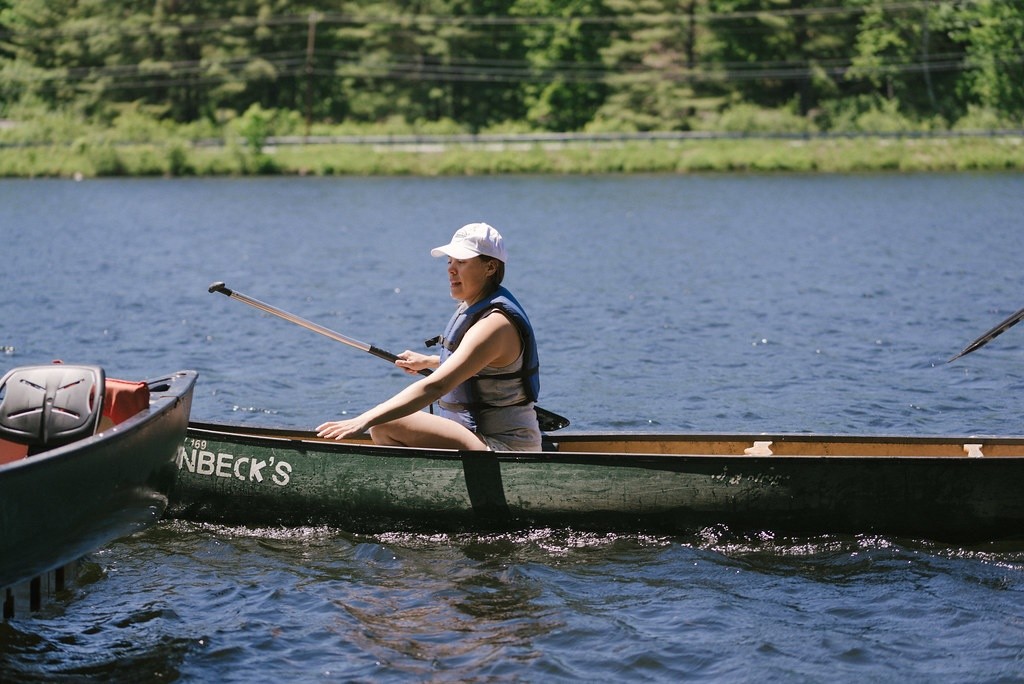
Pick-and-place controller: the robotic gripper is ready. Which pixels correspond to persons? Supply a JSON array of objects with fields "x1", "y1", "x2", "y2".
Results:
[{"x1": 315, "y1": 223, "x2": 544, "y2": 451}]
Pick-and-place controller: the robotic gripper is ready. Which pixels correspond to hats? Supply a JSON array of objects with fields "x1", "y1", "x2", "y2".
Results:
[{"x1": 430, "y1": 221, "x2": 508, "y2": 265}]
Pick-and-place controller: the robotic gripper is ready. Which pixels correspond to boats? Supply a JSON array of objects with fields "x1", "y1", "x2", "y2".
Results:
[
  {"x1": 170, "y1": 423, "x2": 1023, "y2": 555},
  {"x1": 0, "y1": 369, "x2": 199, "y2": 596}
]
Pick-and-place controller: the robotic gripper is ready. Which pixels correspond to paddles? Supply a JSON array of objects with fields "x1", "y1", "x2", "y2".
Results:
[
  {"x1": 208, "y1": 282, "x2": 571, "y2": 432},
  {"x1": 947, "y1": 308, "x2": 1024, "y2": 364}
]
